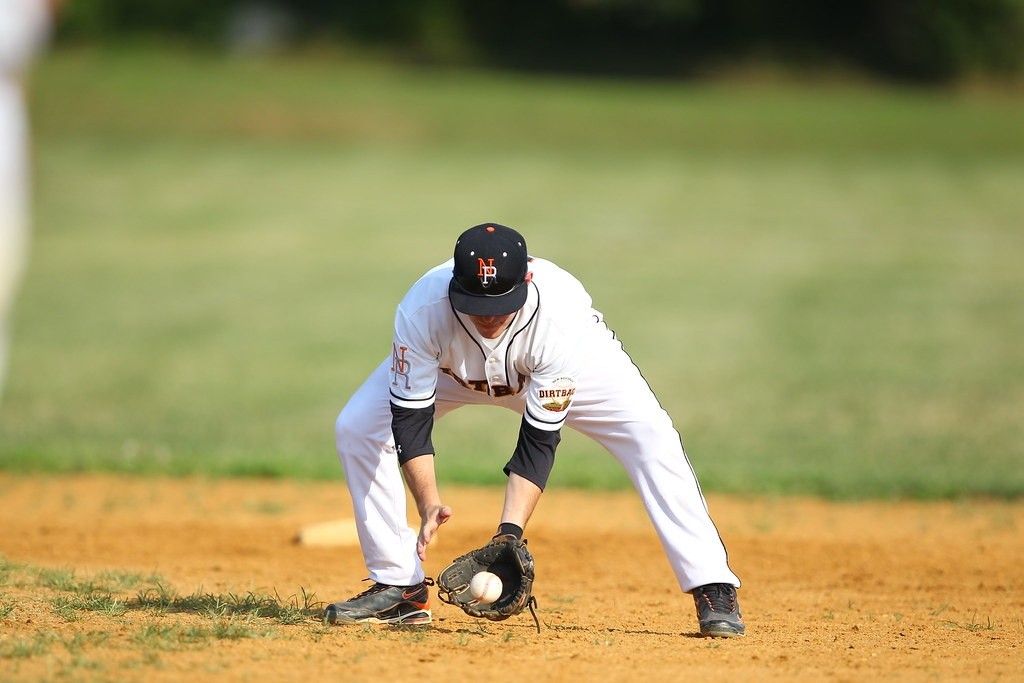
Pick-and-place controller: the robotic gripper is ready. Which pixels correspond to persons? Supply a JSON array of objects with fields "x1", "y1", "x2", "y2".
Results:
[{"x1": 325, "y1": 223, "x2": 746, "y2": 638}]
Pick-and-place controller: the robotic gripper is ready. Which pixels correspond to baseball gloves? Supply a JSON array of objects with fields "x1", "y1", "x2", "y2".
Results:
[{"x1": 436, "y1": 533, "x2": 541, "y2": 633}]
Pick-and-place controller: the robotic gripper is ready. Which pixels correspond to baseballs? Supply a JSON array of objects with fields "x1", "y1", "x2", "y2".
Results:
[{"x1": 470, "y1": 571, "x2": 504, "y2": 604}]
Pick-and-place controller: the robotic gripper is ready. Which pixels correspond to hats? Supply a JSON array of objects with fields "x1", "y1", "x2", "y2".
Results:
[{"x1": 450, "y1": 221, "x2": 528, "y2": 316}]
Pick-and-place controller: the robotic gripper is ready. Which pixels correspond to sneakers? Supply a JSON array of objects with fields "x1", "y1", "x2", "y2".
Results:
[
  {"x1": 323, "y1": 580, "x2": 431, "y2": 627},
  {"x1": 692, "y1": 583, "x2": 745, "y2": 637}
]
